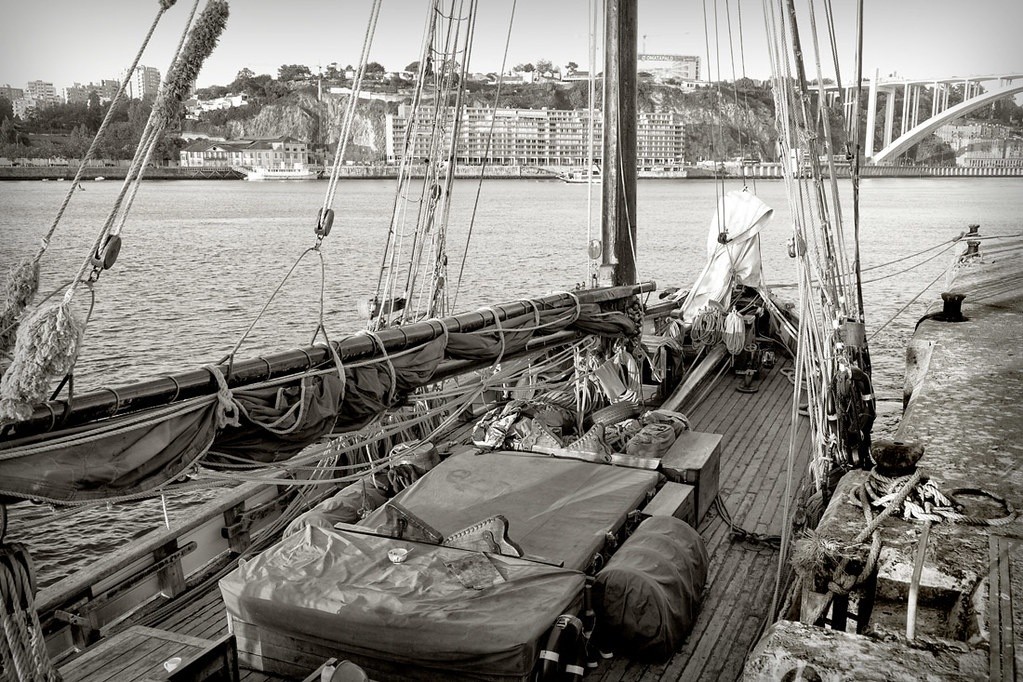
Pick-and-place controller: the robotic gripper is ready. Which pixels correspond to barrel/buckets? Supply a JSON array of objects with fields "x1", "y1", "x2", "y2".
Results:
[{"x1": 390, "y1": 440, "x2": 442, "y2": 477}]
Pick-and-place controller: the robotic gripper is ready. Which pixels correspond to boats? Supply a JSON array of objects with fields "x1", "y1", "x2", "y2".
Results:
[
  {"x1": 242, "y1": 165, "x2": 323, "y2": 182},
  {"x1": 555, "y1": 161, "x2": 602, "y2": 184}
]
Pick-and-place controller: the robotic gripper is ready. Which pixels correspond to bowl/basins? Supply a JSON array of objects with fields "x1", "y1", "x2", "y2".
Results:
[
  {"x1": 387, "y1": 548, "x2": 408, "y2": 562},
  {"x1": 329, "y1": 660, "x2": 369, "y2": 682}
]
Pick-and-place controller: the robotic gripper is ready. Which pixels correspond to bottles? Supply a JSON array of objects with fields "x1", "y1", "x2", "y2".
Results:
[{"x1": 321, "y1": 661, "x2": 336, "y2": 682}]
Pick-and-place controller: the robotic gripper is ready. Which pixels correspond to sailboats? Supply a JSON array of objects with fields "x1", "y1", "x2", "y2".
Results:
[{"x1": 0, "y1": 0, "x2": 920, "y2": 681}]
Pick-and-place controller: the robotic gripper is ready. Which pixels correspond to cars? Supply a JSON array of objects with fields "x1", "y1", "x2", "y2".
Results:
[{"x1": 48, "y1": 158, "x2": 69, "y2": 167}]
